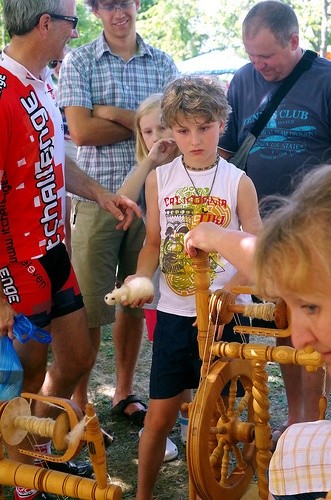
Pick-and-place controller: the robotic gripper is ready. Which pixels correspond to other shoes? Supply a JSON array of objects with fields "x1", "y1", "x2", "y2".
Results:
[
  {"x1": 179, "y1": 410, "x2": 189, "y2": 446},
  {"x1": 270, "y1": 424, "x2": 287, "y2": 452},
  {"x1": 138, "y1": 427, "x2": 178, "y2": 461},
  {"x1": 41, "y1": 459, "x2": 93, "y2": 479}
]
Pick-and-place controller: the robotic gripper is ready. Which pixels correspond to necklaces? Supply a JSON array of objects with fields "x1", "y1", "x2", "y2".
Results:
[{"x1": 181, "y1": 153, "x2": 220, "y2": 213}]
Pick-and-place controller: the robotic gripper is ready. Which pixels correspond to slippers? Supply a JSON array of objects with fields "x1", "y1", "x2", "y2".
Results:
[{"x1": 111, "y1": 394, "x2": 148, "y2": 427}]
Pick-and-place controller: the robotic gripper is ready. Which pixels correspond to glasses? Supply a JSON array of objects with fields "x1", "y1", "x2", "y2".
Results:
[
  {"x1": 97, "y1": 0, "x2": 135, "y2": 12},
  {"x1": 35, "y1": 12, "x2": 78, "y2": 29},
  {"x1": 50, "y1": 60, "x2": 62, "y2": 68}
]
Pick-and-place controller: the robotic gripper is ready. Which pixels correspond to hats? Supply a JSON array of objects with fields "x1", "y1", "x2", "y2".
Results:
[{"x1": 269, "y1": 420, "x2": 331, "y2": 496}]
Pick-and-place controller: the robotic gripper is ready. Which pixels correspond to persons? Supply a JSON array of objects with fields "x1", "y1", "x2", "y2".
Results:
[
  {"x1": 119, "y1": 77, "x2": 264, "y2": 500},
  {"x1": 184, "y1": 164, "x2": 331, "y2": 374},
  {"x1": 54, "y1": 0, "x2": 181, "y2": 452},
  {"x1": 114, "y1": 93, "x2": 194, "y2": 461},
  {"x1": 218, "y1": 0, "x2": 331, "y2": 453},
  {"x1": 0, "y1": 0, "x2": 141, "y2": 500},
  {"x1": 49, "y1": 46, "x2": 78, "y2": 260}
]
